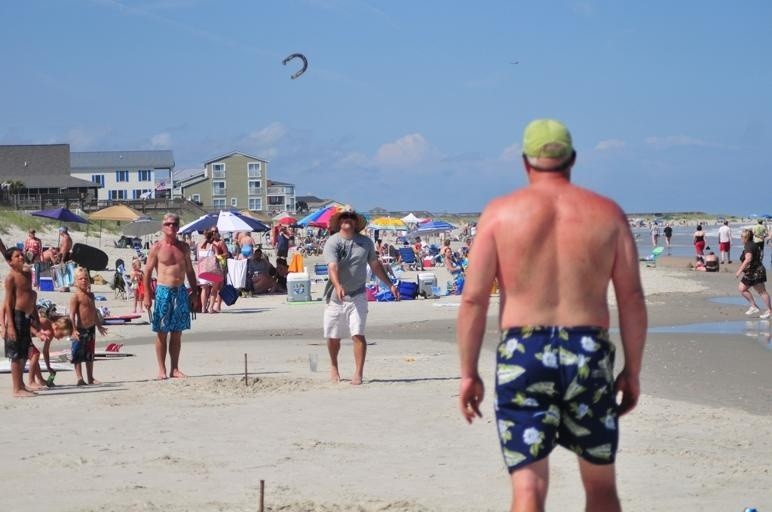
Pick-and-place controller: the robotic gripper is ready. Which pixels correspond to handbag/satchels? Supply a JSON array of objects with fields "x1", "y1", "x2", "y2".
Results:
[
  {"x1": 219, "y1": 284, "x2": 240, "y2": 305},
  {"x1": 197, "y1": 254, "x2": 224, "y2": 282},
  {"x1": 24, "y1": 251, "x2": 34, "y2": 263}
]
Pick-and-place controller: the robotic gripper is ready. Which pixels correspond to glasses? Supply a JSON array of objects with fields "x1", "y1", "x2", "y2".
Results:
[
  {"x1": 212, "y1": 230, "x2": 218, "y2": 233},
  {"x1": 163, "y1": 222, "x2": 180, "y2": 226},
  {"x1": 341, "y1": 212, "x2": 356, "y2": 220}
]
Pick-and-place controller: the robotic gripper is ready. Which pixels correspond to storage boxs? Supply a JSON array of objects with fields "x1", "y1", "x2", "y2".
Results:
[
  {"x1": 362, "y1": 272, "x2": 439, "y2": 303},
  {"x1": 38, "y1": 276, "x2": 55, "y2": 292},
  {"x1": 287, "y1": 271, "x2": 311, "y2": 303}
]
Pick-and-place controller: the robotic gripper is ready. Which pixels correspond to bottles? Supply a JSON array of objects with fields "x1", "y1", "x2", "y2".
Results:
[{"x1": 46, "y1": 372, "x2": 56, "y2": 386}]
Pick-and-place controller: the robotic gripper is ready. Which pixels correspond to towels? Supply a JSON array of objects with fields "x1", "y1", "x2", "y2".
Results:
[{"x1": 227, "y1": 259, "x2": 248, "y2": 291}]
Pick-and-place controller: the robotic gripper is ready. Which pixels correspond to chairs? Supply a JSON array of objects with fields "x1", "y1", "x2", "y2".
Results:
[
  {"x1": 112, "y1": 259, "x2": 213, "y2": 320},
  {"x1": 378, "y1": 240, "x2": 444, "y2": 269},
  {"x1": 225, "y1": 257, "x2": 253, "y2": 298}
]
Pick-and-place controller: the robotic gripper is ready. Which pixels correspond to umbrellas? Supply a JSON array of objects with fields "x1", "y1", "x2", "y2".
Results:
[
  {"x1": 237, "y1": 202, "x2": 368, "y2": 240},
  {"x1": 172, "y1": 208, "x2": 271, "y2": 234},
  {"x1": 30, "y1": 206, "x2": 91, "y2": 250},
  {"x1": 87, "y1": 201, "x2": 142, "y2": 228}
]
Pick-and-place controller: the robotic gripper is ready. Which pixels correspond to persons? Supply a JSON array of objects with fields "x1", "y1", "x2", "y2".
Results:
[
  {"x1": 455, "y1": 119, "x2": 648, "y2": 512},
  {"x1": 321, "y1": 205, "x2": 399, "y2": 383},
  {"x1": 142, "y1": 211, "x2": 199, "y2": 380}
]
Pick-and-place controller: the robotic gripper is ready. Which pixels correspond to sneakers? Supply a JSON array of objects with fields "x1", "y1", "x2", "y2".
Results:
[
  {"x1": 745, "y1": 306, "x2": 758, "y2": 316},
  {"x1": 760, "y1": 309, "x2": 771, "y2": 318}
]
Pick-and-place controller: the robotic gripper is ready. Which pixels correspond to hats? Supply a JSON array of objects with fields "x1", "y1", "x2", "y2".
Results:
[
  {"x1": 522, "y1": 119, "x2": 574, "y2": 159},
  {"x1": 329, "y1": 206, "x2": 367, "y2": 231}
]
